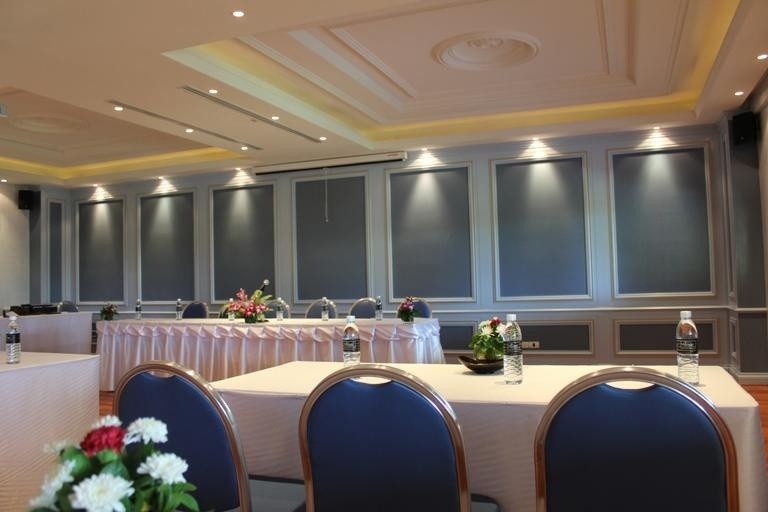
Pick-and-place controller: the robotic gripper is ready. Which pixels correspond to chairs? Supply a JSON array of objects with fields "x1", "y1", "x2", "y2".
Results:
[
  {"x1": 304, "y1": 297, "x2": 338, "y2": 318},
  {"x1": 261, "y1": 297, "x2": 289, "y2": 320},
  {"x1": 112, "y1": 360, "x2": 251, "y2": 511},
  {"x1": 395, "y1": 299, "x2": 431, "y2": 318},
  {"x1": 299, "y1": 363, "x2": 470, "y2": 512},
  {"x1": 533, "y1": 366, "x2": 739, "y2": 512},
  {"x1": 58, "y1": 300, "x2": 78, "y2": 312},
  {"x1": 349, "y1": 297, "x2": 376, "y2": 318},
  {"x1": 183, "y1": 301, "x2": 209, "y2": 317}
]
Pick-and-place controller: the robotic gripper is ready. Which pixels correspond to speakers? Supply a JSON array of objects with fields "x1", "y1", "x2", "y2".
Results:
[
  {"x1": 18, "y1": 190, "x2": 33, "y2": 210},
  {"x1": 733, "y1": 113, "x2": 762, "y2": 146}
]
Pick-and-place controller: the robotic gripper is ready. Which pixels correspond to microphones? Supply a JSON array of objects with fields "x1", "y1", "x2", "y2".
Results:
[{"x1": 256, "y1": 279, "x2": 271, "y2": 292}]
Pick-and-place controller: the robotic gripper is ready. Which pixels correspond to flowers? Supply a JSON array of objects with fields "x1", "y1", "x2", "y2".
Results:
[
  {"x1": 100, "y1": 303, "x2": 118, "y2": 316},
  {"x1": 398, "y1": 297, "x2": 414, "y2": 314},
  {"x1": 466, "y1": 317, "x2": 506, "y2": 361},
  {"x1": 226, "y1": 288, "x2": 272, "y2": 317},
  {"x1": 28, "y1": 415, "x2": 199, "y2": 512}
]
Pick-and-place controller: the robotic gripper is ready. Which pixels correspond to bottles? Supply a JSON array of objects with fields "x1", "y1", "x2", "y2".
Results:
[
  {"x1": 228, "y1": 298, "x2": 234, "y2": 320},
  {"x1": 503, "y1": 313, "x2": 523, "y2": 384},
  {"x1": 176, "y1": 299, "x2": 182, "y2": 320},
  {"x1": 342, "y1": 316, "x2": 360, "y2": 366},
  {"x1": 676, "y1": 310, "x2": 698, "y2": 386},
  {"x1": 135, "y1": 299, "x2": 142, "y2": 319},
  {"x1": 375, "y1": 296, "x2": 383, "y2": 320},
  {"x1": 276, "y1": 297, "x2": 283, "y2": 321},
  {"x1": 6, "y1": 315, "x2": 21, "y2": 363},
  {"x1": 321, "y1": 297, "x2": 329, "y2": 321}
]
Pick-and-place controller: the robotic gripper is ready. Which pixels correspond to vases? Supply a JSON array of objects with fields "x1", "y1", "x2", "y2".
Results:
[
  {"x1": 104, "y1": 315, "x2": 113, "y2": 321},
  {"x1": 244, "y1": 313, "x2": 257, "y2": 323},
  {"x1": 399, "y1": 312, "x2": 412, "y2": 322},
  {"x1": 459, "y1": 356, "x2": 504, "y2": 374}
]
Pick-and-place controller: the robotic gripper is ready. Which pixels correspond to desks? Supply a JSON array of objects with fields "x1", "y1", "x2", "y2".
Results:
[
  {"x1": 1, "y1": 351, "x2": 100, "y2": 511},
  {"x1": 199, "y1": 360, "x2": 766, "y2": 512},
  {"x1": 95, "y1": 319, "x2": 447, "y2": 392},
  {"x1": 0, "y1": 311, "x2": 92, "y2": 354}
]
